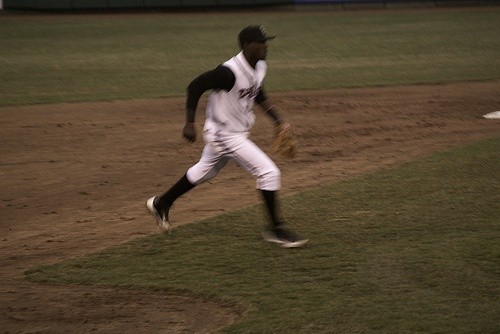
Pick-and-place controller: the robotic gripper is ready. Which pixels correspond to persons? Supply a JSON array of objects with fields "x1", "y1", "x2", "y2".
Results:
[{"x1": 145, "y1": 25, "x2": 309, "y2": 250}]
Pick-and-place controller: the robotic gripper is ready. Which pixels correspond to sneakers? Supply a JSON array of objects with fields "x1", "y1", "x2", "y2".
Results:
[
  {"x1": 145, "y1": 195, "x2": 173, "y2": 233},
  {"x1": 262, "y1": 224, "x2": 308, "y2": 248}
]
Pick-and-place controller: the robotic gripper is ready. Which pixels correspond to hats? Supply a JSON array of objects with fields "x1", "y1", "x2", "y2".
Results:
[{"x1": 238, "y1": 25, "x2": 277, "y2": 46}]
